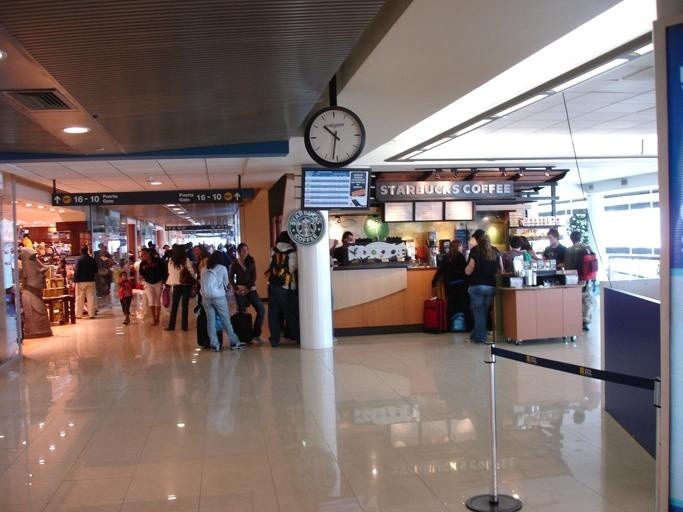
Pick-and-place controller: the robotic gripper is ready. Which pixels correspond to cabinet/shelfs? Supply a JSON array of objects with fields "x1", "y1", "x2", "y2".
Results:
[{"x1": 502, "y1": 287, "x2": 583, "y2": 345}]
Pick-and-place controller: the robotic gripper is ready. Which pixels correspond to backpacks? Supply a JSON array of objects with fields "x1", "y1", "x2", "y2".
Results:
[
  {"x1": 264, "y1": 247, "x2": 295, "y2": 291},
  {"x1": 449, "y1": 312, "x2": 467, "y2": 332},
  {"x1": 200, "y1": 265, "x2": 223, "y2": 298},
  {"x1": 578, "y1": 247, "x2": 598, "y2": 280}
]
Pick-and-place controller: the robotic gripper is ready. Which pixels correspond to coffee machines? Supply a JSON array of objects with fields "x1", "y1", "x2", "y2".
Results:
[{"x1": 427, "y1": 231, "x2": 439, "y2": 267}]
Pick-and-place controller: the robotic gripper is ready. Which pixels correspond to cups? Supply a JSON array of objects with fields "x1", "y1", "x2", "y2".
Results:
[{"x1": 525, "y1": 269, "x2": 536, "y2": 286}]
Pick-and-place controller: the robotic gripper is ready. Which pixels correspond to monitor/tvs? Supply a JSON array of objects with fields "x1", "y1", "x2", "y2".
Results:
[{"x1": 301, "y1": 167, "x2": 372, "y2": 210}]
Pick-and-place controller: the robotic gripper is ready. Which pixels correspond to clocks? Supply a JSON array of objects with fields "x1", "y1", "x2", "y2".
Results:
[{"x1": 305, "y1": 105, "x2": 364, "y2": 166}]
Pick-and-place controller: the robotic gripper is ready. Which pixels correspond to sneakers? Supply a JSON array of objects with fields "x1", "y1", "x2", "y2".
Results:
[
  {"x1": 123, "y1": 317, "x2": 130, "y2": 325},
  {"x1": 231, "y1": 342, "x2": 247, "y2": 350},
  {"x1": 212, "y1": 345, "x2": 221, "y2": 352}
]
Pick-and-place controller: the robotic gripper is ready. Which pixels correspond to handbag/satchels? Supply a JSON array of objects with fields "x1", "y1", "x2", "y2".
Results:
[
  {"x1": 179, "y1": 262, "x2": 195, "y2": 285},
  {"x1": 163, "y1": 288, "x2": 170, "y2": 307}
]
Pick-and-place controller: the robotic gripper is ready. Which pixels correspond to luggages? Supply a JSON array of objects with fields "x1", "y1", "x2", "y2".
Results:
[
  {"x1": 423, "y1": 297, "x2": 449, "y2": 333},
  {"x1": 230, "y1": 290, "x2": 253, "y2": 343},
  {"x1": 196, "y1": 315, "x2": 223, "y2": 348}
]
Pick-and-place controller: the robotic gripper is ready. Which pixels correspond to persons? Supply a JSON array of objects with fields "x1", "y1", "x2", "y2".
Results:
[
  {"x1": 329, "y1": 231, "x2": 354, "y2": 268},
  {"x1": 66, "y1": 231, "x2": 300, "y2": 352},
  {"x1": 431, "y1": 227, "x2": 598, "y2": 345}
]
